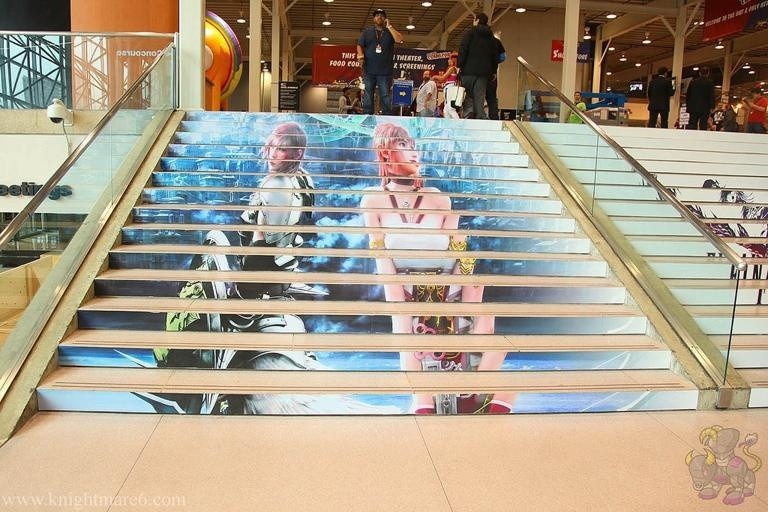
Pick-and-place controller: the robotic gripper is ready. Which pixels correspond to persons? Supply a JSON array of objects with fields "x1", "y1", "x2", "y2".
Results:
[
  {"x1": 361, "y1": 123, "x2": 518, "y2": 414},
  {"x1": 338, "y1": 88, "x2": 364, "y2": 114},
  {"x1": 455, "y1": 12, "x2": 498, "y2": 119},
  {"x1": 416, "y1": 70, "x2": 437, "y2": 116},
  {"x1": 432, "y1": 59, "x2": 457, "y2": 86},
  {"x1": 356, "y1": 8, "x2": 404, "y2": 114},
  {"x1": 705, "y1": 87, "x2": 768, "y2": 134},
  {"x1": 564, "y1": 91, "x2": 587, "y2": 124},
  {"x1": 486, "y1": 24, "x2": 506, "y2": 119},
  {"x1": 165, "y1": 122, "x2": 315, "y2": 414},
  {"x1": 528, "y1": 95, "x2": 547, "y2": 122},
  {"x1": 685, "y1": 65, "x2": 715, "y2": 130},
  {"x1": 647, "y1": 66, "x2": 676, "y2": 127}
]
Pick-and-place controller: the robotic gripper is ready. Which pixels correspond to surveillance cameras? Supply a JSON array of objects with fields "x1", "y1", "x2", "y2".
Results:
[{"x1": 47, "y1": 104, "x2": 65, "y2": 125}]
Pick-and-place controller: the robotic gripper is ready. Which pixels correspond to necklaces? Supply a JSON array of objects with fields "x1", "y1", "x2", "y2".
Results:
[
  {"x1": 373, "y1": 28, "x2": 386, "y2": 54},
  {"x1": 419, "y1": 79, "x2": 430, "y2": 91},
  {"x1": 386, "y1": 172, "x2": 417, "y2": 189}
]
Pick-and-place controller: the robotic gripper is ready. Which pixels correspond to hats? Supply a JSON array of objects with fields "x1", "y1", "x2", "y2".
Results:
[
  {"x1": 343, "y1": 87, "x2": 350, "y2": 92},
  {"x1": 751, "y1": 88, "x2": 760, "y2": 93},
  {"x1": 373, "y1": 9, "x2": 386, "y2": 17}
]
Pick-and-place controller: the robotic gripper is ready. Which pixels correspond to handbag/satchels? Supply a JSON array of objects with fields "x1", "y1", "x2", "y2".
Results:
[{"x1": 449, "y1": 86, "x2": 466, "y2": 109}]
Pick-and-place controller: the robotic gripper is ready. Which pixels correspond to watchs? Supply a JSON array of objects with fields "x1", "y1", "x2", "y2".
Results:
[{"x1": 386, "y1": 24, "x2": 393, "y2": 29}]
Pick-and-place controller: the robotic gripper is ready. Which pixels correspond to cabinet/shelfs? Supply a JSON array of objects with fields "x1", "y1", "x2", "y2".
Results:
[{"x1": 278, "y1": 82, "x2": 300, "y2": 113}]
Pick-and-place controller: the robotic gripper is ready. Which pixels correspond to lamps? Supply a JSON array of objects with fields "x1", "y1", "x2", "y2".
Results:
[{"x1": 44, "y1": 99, "x2": 73, "y2": 159}]
